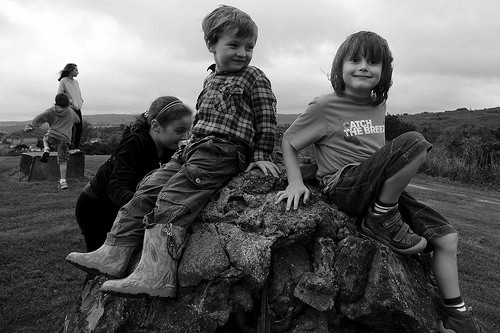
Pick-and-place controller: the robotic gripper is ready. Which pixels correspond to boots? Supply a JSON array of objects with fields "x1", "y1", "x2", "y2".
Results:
[
  {"x1": 66, "y1": 243, "x2": 139, "y2": 279},
  {"x1": 99, "y1": 223, "x2": 189, "y2": 298}
]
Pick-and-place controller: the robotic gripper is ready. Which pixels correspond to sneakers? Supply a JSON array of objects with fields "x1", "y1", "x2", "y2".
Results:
[
  {"x1": 361, "y1": 207, "x2": 427, "y2": 254},
  {"x1": 437, "y1": 307, "x2": 490, "y2": 333}
]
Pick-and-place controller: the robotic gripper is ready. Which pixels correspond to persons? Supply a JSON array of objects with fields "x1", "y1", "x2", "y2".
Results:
[
  {"x1": 57, "y1": 63, "x2": 84, "y2": 151},
  {"x1": 64, "y1": 4, "x2": 282, "y2": 298},
  {"x1": 23, "y1": 92, "x2": 80, "y2": 189},
  {"x1": 274, "y1": 30, "x2": 493, "y2": 333},
  {"x1": 75, "y1": 95, "x2": 193, "y2": 253}
]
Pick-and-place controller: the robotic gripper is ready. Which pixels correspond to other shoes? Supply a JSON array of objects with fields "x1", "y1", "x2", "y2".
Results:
[
  {"x1": 58, "y1": 180, "x2": 68, "y2": 189},
  {"x1": 40, "y1": 148, "x2": 50, "y2": 163}
]
